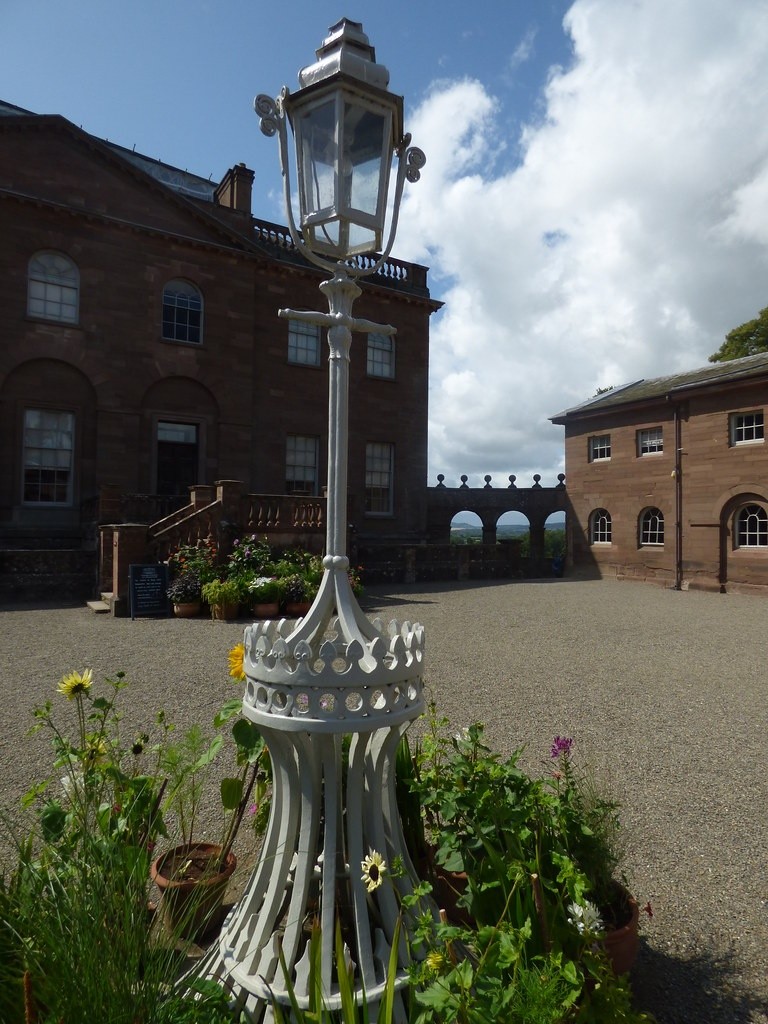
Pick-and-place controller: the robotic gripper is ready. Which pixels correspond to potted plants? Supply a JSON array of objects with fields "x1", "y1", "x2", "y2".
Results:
[{"x1": 201, "y1": 578, "x2": 249, "y2": 622}]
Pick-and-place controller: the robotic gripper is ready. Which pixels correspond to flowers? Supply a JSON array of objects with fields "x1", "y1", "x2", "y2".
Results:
[
  {"x1": 162, "y1": 534, "x2": 366, "y2": 606},
  {"x1": 29, "y1": 645, "x2": 625, "y2": 941}
]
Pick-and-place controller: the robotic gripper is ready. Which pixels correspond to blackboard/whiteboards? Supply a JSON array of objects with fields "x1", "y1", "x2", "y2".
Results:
[{"x1": 128, "y1": 563, "x2": 168, "y2": 614}]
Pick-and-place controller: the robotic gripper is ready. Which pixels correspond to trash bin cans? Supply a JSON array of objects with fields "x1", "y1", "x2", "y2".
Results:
[{"x1": 498, "y1": 539, "x2": 523, "y2": 562}]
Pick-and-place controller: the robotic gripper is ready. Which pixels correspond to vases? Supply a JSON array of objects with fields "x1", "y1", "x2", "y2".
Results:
[
  {"x1": 173, "y1": 602, "x2": 200, "y2": 617},
  {"x1": 571, "y1": 878, "x2": 639, "y2": 979},
  {"x1": 252, "y1": 603, "x2": 279, "y2": 618},
  {"x1": 151, "y1": 843, "x2": 238, "y2": 939},
  {"x1": 284, "y1": 602, "x2": 312, "y2": 617}
]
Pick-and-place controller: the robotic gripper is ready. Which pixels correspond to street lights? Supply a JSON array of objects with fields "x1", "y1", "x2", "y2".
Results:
[{"x1": 168, "y1": 20, "x2": 427, "y2": 1024}]
